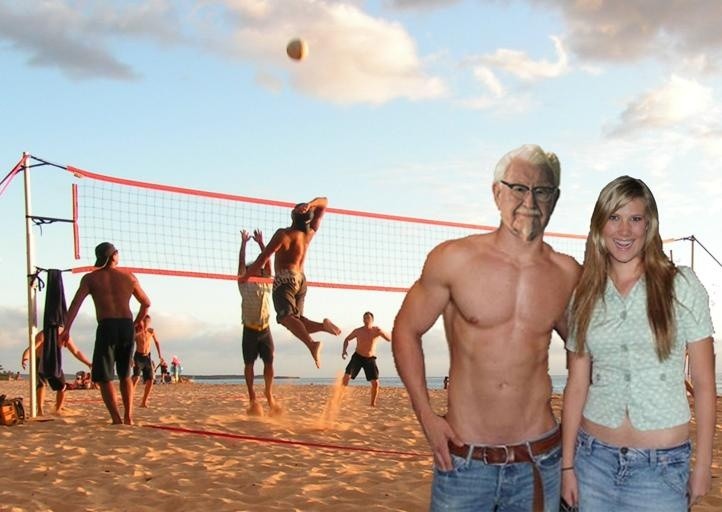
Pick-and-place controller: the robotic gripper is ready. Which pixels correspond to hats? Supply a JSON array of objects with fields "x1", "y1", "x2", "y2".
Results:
[{"x1": 94, "y1": 242, "x2": 117, "y2": 268}]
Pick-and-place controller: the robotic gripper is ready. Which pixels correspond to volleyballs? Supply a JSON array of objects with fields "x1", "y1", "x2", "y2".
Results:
[{"x1": 286, "y1": 38, "x2": 308, "y2": 62}]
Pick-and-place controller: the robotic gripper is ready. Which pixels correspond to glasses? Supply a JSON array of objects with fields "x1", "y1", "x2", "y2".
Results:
[{"x1": 500, "y1": 180, "x2": 558, "y2": 203}]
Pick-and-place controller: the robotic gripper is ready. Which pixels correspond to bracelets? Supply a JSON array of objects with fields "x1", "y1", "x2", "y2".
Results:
[{"x1": 561, "y1": 466, "x2": 575, "y2": 472}]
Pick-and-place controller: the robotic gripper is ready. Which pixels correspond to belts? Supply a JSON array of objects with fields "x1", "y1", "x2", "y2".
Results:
[{"x1": 447, "y1": 426, "x2": 562, "y2": 512}]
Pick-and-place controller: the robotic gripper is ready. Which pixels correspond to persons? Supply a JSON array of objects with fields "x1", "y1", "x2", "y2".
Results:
[
  {"x1": 150, "y1": 356, "x2": 184, "y2": 385},
  {"x1": 233, "y1": 195, "x2": 342, "y2": 369},
  {"x1": 58, "y1": 241, "x2": 150, "y2": 425},
  {"x1": 342, "y1": 311, "x2": 391, "y2": 407},
  {"x1": 14, "y1": 371, "x2": 22, "y2": 381},
  {"x1": 75, "y1": 374, "x2": 83, "y2": 388},
  {"x1": 83, "y1": 372, "x2": 100, "y2": 390},
  {"x1": 391, "y1": 143, "x2": 585, "y2": 511},
  {"x1": 559, "y1": 174, "x2": 717, "y2": 512},
  {"x1": 23, "y1": 324, "x2": 95, "y2": 418},
  {"x1": 237, "y1": 226, "x2": 276, "y2": 415},
  {"x1": 132, "y1": 314, "x2": 163, "y2": 408}
]
